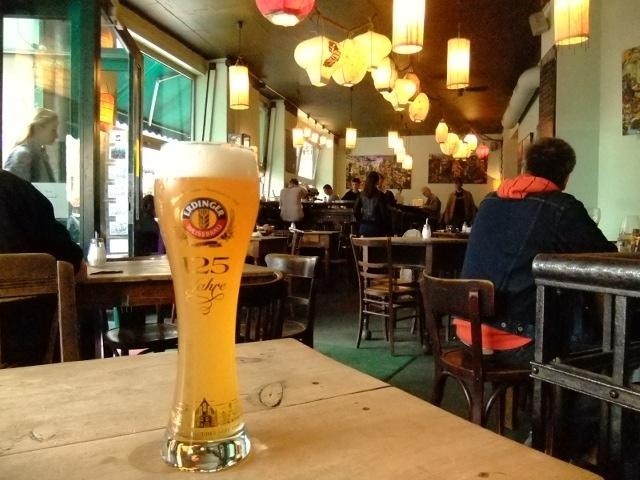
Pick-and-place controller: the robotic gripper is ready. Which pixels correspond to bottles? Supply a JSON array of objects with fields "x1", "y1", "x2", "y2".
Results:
[
  {"x1": 422, "y1": 224, "x2": 431, "y2": 240},
  {"x1": 461, "y1": 222, "x2": 467, "y2": 232},
  {"x1": 87, "y1": 238, "x2": 107, "y2": 267}
]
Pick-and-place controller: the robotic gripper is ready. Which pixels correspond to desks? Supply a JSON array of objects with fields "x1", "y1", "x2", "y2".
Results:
[{"x1": 1, "y1": 337, "x2": 603, "y2": 480}]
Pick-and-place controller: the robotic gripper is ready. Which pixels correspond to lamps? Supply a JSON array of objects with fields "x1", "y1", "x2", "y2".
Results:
[
  {"x1": 345, "y1": 85, "x2": 358, "y2": 150},
  {"x1": 228, "y1": 21, "x2": 252, "y2": 112}
]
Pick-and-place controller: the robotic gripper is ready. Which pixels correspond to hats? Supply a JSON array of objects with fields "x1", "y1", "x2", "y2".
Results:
[{"x1": 421, "y1": 186, "x2": 428, "y2": 194}]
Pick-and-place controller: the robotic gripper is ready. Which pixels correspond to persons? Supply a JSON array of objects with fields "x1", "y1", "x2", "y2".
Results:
[
  {"x1": 453, "y1": 137, "x2": 618, "y2": 467},
  {"x1": 5, "y1": 107, "x2": 60, "y2": 183},
  {"x1": 1, "y1": 169, "x2": 92, "y2": 368},
  {"x1": 134, "y1": 193, "x2": 165, "y2": 254},
  {"x1": 443, "y1": 177, "x2": 477, "y2": 226},
  {"x1": 420, "y1": 187, "x2": 442, "y2": 227},
  {"x1": 278, "y1": 172, "x2": 400, "y2": 288}
]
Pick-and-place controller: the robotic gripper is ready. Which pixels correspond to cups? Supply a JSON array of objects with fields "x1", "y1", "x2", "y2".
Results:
[
  {"x1": 155, "y1": 142, "x2": 260, "y2": 472},
  {"x1": 617, "y1": 216, "x2": 640, "y2": 255},
  {"x1": 589, "y1": 207, "x2": 600, "y2": 225}
]
